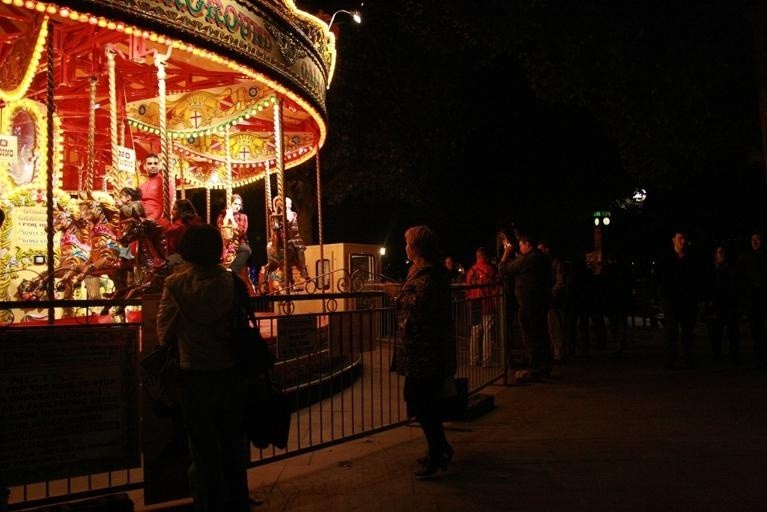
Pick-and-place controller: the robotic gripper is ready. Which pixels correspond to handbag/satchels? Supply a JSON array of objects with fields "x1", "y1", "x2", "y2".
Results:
[{"x1": 227, "y1": 270, "x2": 277, "y2": 383}]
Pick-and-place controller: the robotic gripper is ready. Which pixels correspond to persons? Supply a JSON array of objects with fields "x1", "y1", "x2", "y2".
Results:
[
  {"x1": 108, "y1": 154, "x2": 202, "y2": 290},
  {"x1": 446, "y1": 228, "x2": 764, "y2": 382},
  {"x1": 14, "y1": 154, "x2": 310, "y2": 320},
  {"x1": 151, "y1": 224, "x2": 291, "y2": 511},
  {"x1": 384, "y1": 226, "x2": 458, "y2": 476},
  {"x1": 217, "y1": 194, "x2": 252, "y2": 274},
  {"x1": 266, "y1": 198, "x2": 308, "y2": 281}
]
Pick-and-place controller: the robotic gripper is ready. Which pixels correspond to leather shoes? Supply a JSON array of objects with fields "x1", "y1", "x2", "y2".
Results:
[
  {"x1": 416, "y1": 454, "x2": 448, "y2": 478},
  {"x1": 418, "y1": 445, "x2": 452, "y2": 464}
]
